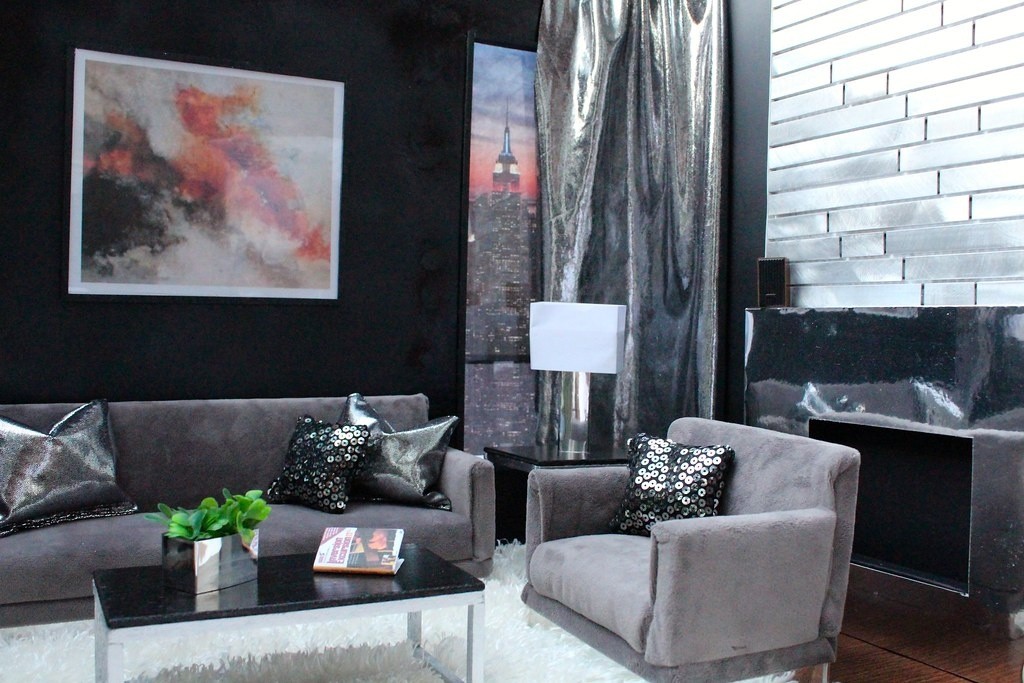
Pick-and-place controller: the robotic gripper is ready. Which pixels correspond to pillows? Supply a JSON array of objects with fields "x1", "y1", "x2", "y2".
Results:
[
  {"x1": 336, "y1": 393, "x2": 459, "y2": 513},
  {"x1": 267, "y1": 415, "x2": 372, "y2": 514},
  {"x1": 607, "y1": 432, "x2": 736, "y2": 537},
  {"x1": 0, "y1": 393, "x2": 138, "y2": 539}
]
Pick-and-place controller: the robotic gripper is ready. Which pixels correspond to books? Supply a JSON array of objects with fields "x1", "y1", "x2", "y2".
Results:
[{"x1": 313, "y1": 526, "x2": 404, "y2": 577}]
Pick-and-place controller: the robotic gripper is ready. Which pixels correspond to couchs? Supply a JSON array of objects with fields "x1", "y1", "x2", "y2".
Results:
[
  {"x1": 0, "y1": 392, "x2": 497, "y2": 631},
  {"x1": 524, "y1": 416, "x2": 862, "y2": 683}
]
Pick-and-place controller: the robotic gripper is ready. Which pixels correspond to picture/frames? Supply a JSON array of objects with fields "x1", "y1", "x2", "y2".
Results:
[{"x1": 55, "y1": 47, "x2": 346, "y2": 299}]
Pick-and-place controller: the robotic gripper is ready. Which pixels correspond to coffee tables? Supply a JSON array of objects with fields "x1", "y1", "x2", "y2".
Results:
[{"x1": 91, "y1": 542, "x2": 488, "y2": 683}]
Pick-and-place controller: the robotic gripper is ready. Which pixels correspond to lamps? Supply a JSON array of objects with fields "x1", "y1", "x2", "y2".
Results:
[{"x1": 528, "y1": 301, "x2": 624, "y2": 438}]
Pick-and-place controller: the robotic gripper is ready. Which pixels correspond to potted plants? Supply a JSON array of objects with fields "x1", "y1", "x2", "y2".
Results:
[{"x1": 144, "y1": 486, "x2": 273, "y2": 595}]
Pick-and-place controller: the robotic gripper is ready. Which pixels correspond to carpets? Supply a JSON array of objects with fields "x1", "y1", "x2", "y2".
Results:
[{"x1": 0, "y1": 537, "x2": 801, "y2": 683}]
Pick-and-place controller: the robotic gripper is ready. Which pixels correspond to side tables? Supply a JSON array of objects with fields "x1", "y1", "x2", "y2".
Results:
[{"x1": 481, "y1": 439, "x2": 625, "y2": 544}]
